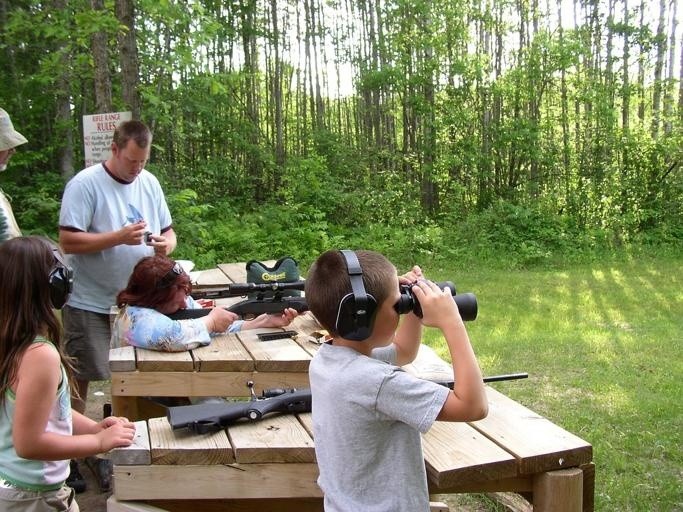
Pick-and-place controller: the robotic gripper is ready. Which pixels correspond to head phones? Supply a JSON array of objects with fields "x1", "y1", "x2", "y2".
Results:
[
  {"x1": 334, "y1": 251, "x2": 378, "y2": 340},
  {"x1": 43, "y1": 243, "x2": 74, "y2": 308}
]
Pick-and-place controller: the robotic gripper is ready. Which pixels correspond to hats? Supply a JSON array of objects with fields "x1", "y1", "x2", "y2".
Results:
[{"x1": 0, "y1": 107, "x2": 29, "y2": 152}]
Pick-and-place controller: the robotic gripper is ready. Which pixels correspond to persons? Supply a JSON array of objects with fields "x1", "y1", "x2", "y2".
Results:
[
  {"x1": 0, "y1": 108, "x2": 30, "y2": 244},
  {"x1": 305, "y1": 249, "x2": 490, "y2": 512},
  {"x1": 110, "y1": 254, "x2": 299, "y2": 405},
  {"x1": 0, "y1": 235, "x2": 136, "y2": 512},
  {"x1": 57, "y1": 119, "x2": 179, "y2": 418}
]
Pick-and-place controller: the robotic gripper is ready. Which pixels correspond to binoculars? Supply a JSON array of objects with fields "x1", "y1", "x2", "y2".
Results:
[{"x1": 395, "y1": 280, "x2": 478, "y2": 321}]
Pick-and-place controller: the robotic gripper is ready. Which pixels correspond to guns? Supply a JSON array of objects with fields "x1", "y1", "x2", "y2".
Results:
[
  {"x1": 165, "y1": 281, "x2": 308, "y2": 320},
  {"x1": 166, "y1": 373, "x2": 528, "y2": 434}
]
